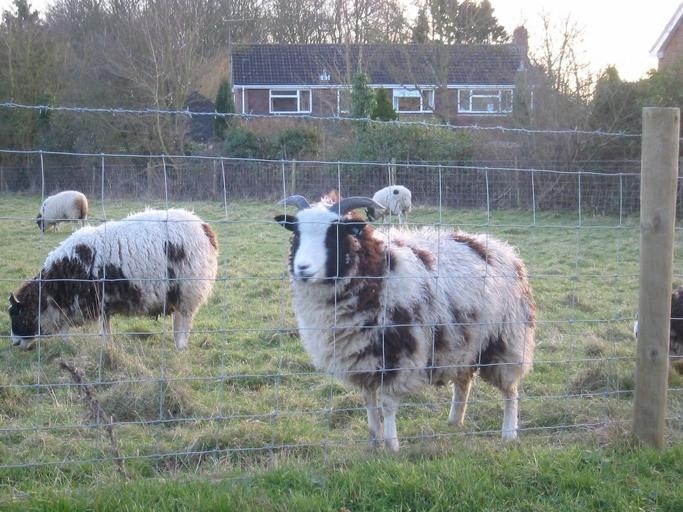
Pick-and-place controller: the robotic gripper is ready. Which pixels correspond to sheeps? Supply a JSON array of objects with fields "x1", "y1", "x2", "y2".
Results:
[
  {"x1": 633, "y1": 282, "x2": 683, "y2": 380},
  {"x1": 35, "y1": 189, "x2": 90, "y2": 232},
  {"x1": 6, "y1": 205, "x2": 220, "y2": 352},
  {"x1": 272, "y1": 187, "x2": 539, "y2": 455},
  {"x1": 372, "y1": 184, "x2": 412, "y2": 232}
]
